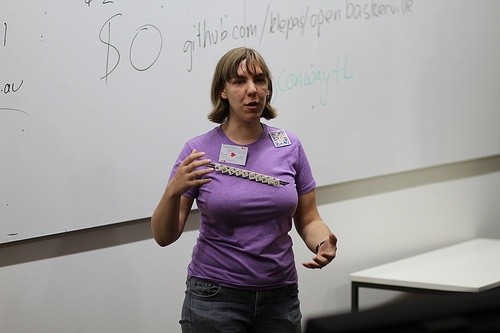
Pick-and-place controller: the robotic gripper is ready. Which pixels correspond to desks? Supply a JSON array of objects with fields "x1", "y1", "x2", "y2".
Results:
[{"x1": 352, "y1": 237, "x2": 500, "y2": 315}]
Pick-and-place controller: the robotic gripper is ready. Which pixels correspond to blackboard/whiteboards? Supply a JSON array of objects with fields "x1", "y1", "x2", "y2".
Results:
[{"x1": 0, "y1": 0, "x2": 500, "y2": 245}]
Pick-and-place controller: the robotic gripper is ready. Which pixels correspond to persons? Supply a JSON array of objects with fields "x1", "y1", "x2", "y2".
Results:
[{"x1": 150, "y1": 46, "x2": 337, "y2": 333}]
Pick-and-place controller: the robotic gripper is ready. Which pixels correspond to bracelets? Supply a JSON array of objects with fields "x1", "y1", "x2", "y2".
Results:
[{"x1": 316, "y1": 241, "x2": 324, "y2": 252}]
